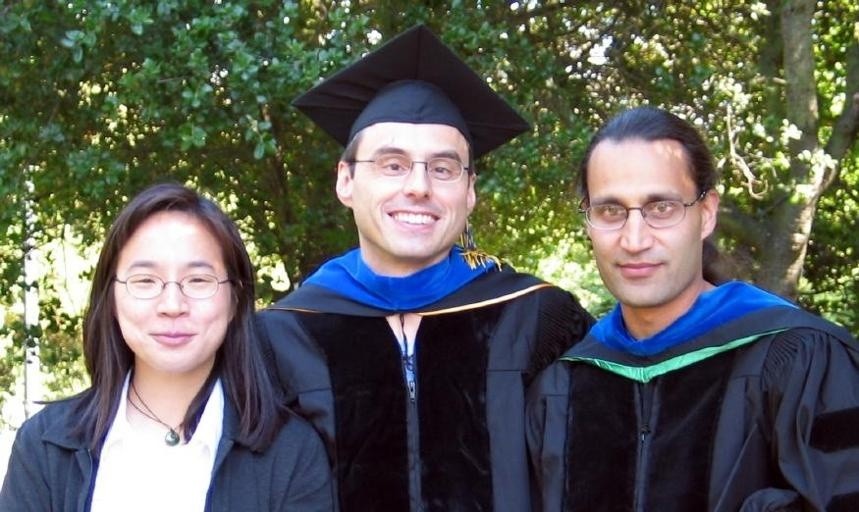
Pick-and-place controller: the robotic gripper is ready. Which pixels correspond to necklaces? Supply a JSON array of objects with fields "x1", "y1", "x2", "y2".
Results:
[{"x1": 127, "y1": 381, "x2": 187, "y2": 447}]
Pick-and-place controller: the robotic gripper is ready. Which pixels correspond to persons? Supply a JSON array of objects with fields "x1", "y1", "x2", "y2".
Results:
[
  {"x1": 253, "y1": 22, "x2": 600, "y2": 510},
  {"x1": 0, "y1": 183, "x2": 337, "y2": 511},
  {"x1": 523, "y1": 105, "x2": 859, "y2": 511}
]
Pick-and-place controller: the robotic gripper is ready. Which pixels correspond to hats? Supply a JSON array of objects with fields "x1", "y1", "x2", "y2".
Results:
[{"x1": 289, "y1": 21, "x2": 533, "y2": 161}]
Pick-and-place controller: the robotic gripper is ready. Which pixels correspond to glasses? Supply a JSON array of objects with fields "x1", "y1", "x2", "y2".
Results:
[
  {"x1": 576, "y1": 187, "x2": 708, "y2": 232},
  {"x1": 110, "y1": 272, "x2": 236, "y2": 300},
  {"x1": 344, "y1": 155, "x2": 475, "y2": 185}
]
